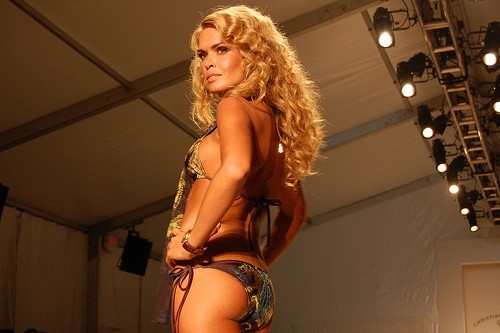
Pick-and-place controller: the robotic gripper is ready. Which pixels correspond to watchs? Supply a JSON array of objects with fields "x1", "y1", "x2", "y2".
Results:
[{"x1": 181, "y1": 229, "x2": 208, "y2": 255}]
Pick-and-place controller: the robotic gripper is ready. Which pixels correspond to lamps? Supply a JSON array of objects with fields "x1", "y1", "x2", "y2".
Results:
[
  {"x1": 417, "y1": 105, "x2": 453, "y2": 139},
  {"x1": 447, "y1": 155, "x2": 467, "y2": 195},
  {"x1": 372, "y1": 6, "x2": 418, "y2": 48},
  {"x1": 466, "y1": 206, "x2": 490, "y2": 233},
  {"x1": 396, "y1": 53, "x2": 436, "y2": 98},
  {"x1": 457, "y1": 185, "x2": 484, "y2": 217},
  {"x1": 431, "y1": 138, "x2": 464, "y2": 173},
  {"x1": 462, "y1": 21, "x2": 500, "y2": 74}
]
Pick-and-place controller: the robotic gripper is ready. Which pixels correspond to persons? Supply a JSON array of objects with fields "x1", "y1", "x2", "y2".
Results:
[{"x1": 166, "y1": 4, "x2": 328, "y2": 333}]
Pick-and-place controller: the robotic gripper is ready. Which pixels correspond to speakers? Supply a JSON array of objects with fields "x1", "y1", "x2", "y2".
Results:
[
  {"x1": 0, "y1": 183, "x2": 9, "y2": 220},
  {"x1": 118, "y1": 234, "x2": 153, "y2": 276}
]
